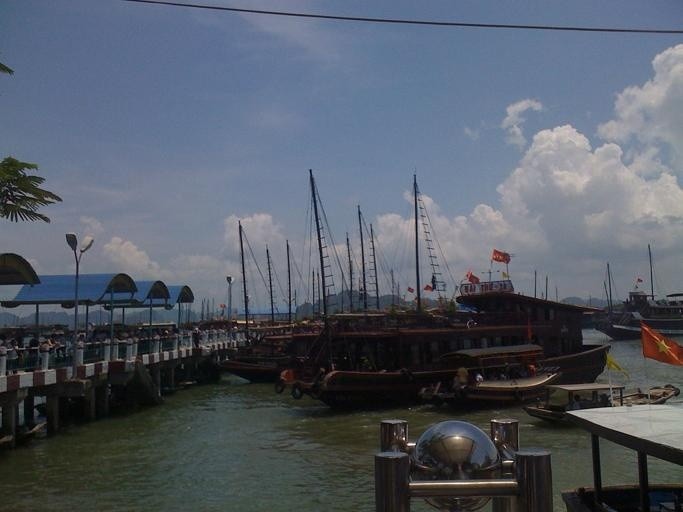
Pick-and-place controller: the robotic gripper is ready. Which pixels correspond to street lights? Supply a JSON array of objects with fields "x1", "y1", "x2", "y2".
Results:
[{"x1": 65, "y1": 232, "x2": 94, "y2": 380}]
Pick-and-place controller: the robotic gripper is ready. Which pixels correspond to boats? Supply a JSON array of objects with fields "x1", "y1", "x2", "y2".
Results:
[
  {"x1": 523, "y1": 383, "x2": 680, "y2": 426},
  {"x1": 554, "y1": 405, "x2": 682, "y2": 512}
]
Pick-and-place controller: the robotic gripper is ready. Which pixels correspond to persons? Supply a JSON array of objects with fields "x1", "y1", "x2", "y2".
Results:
[
  {"x1": 527, "y1": 362, "x2": 537, "y2": 377},
  {"x1": 597, "y1": 392, "x2": 612, "y2": 406},
  {"x1": 571, "y1": 394, "x2": 582, "y2": 409},
  {"x1": 501, "y1": 364, "x2": 510, "y2": 380},
  {"x1": 472, "y1": 369, "x2": 482, "y2": 384},
  {"x1": 2, "y1": 325, "x2": 239, "y2": 376}
]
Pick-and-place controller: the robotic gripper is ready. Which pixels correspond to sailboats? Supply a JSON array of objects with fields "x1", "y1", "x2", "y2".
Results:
[
  {"x1": 214, "y1": 168, "x2": 612, "y2": 410},
  {"x1": 590, "y1": 243, "x2": 683, "y2": 342}
]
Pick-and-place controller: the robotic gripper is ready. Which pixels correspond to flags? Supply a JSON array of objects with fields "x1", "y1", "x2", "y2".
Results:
[
  {"x1": 636, "y1": 277, "x2": 646, "y2": 284},
  {"x1": 500, "y1": 271, "x2": 509, "y2": 279},
  {"x1": 219, "y1": 303, "x2": 226, "y2": 308},
  {"x1": 467, "y1": 270, "x2": 481, "y2": 284},
  {"x1": 406, "y1": 287, "x2": 413, "y2": 293},
  {"x1": 423, "y1": 284, "x2": 434, "y2": 293},
  {"x1": 640, "y1": 320, "x2": 681, "y2": 366},
  {"x1": 490, "y1": 249, "x2": 511, "y2": 264}
]
